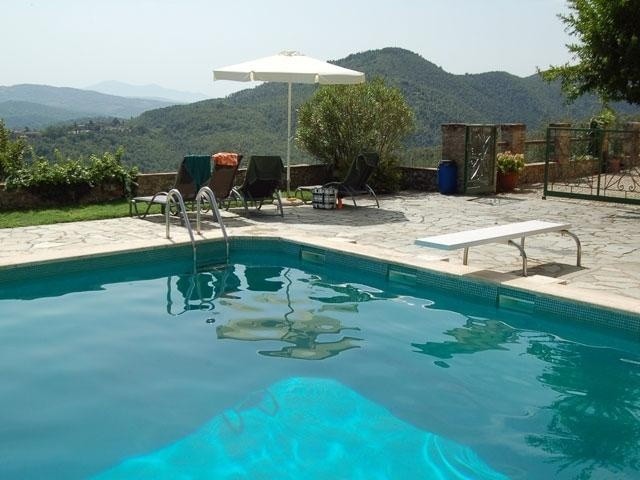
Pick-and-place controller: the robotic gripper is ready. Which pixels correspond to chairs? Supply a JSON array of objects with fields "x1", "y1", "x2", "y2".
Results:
[
  {"x1": 128, "y1": 152, "x2": 286, "y2": 220},
  {"x1": 294, "y1": 151, "x2": 380, "y2": 209}
]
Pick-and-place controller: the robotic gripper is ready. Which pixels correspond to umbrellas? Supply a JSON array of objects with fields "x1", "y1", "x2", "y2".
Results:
[{"x1": 213, "y1": 52, "x2": 366, "y2": 198}]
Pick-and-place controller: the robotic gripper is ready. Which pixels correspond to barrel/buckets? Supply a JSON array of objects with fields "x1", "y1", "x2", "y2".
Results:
[
  {"x1": 313, "y1": 188, "x2": 338, "y2": 209},
  {"x1": 438, "y1": 160, "x2": 457, "y2": 195}
]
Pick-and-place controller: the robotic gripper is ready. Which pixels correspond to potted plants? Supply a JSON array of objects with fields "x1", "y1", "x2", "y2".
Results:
[{"x1": 496, "y1": 149, "x2": 525, "y2": 192}]
